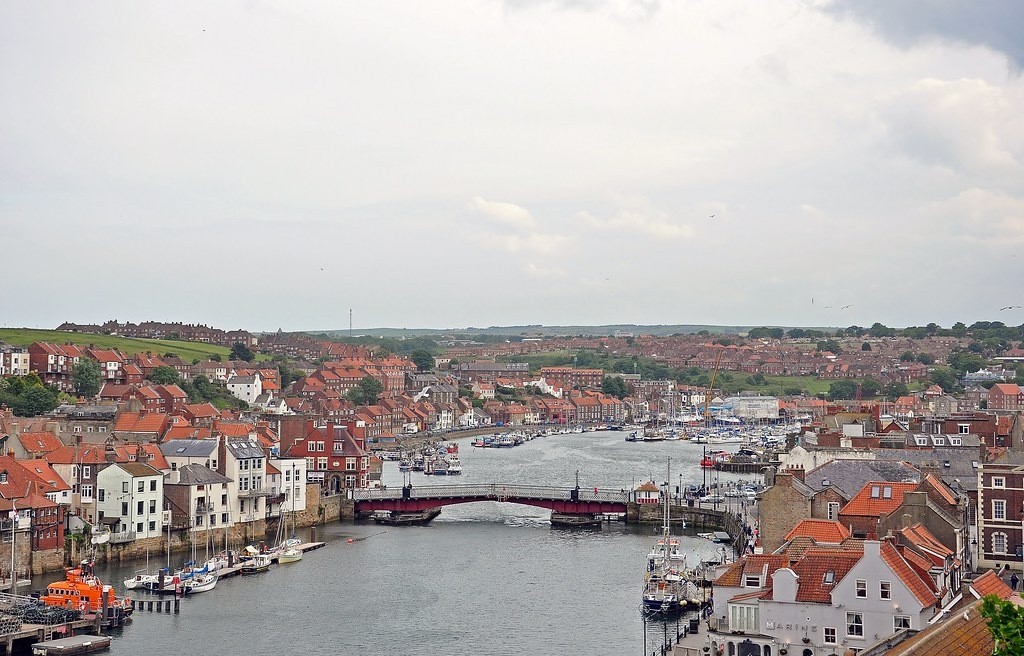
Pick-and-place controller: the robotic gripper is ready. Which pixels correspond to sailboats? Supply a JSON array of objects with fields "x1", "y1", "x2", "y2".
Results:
[
  {"x1": 641, "y1": 452, "x2": 691, "y2": 615},
  {"x1": 39, "y1": 505, "x2": 305, "y2": 622}
]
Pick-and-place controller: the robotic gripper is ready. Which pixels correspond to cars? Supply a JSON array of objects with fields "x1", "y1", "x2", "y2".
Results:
[
  {"x1": 699, "y1": 482, "x2": 762, "y2": 503},
  {"x1": 395, "y1": 416, "x2": 615, "y2": 440}
]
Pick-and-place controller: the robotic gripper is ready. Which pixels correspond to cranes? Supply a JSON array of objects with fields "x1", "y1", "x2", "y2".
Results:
[{"x1": 780, "y1": 349, "x2": 818, "y2": 397}]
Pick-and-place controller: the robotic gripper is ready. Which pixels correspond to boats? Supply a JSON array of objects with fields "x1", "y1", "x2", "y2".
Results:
[
  {"x1": 471, "y1": 424, "x2": 624, "y2": 448},
  {"x1": 397, "y1": 440, "x2": 462, "y2": 476},
  {"x1": 624, "y1": 423, "x2": 805, "y2": 470}
]
